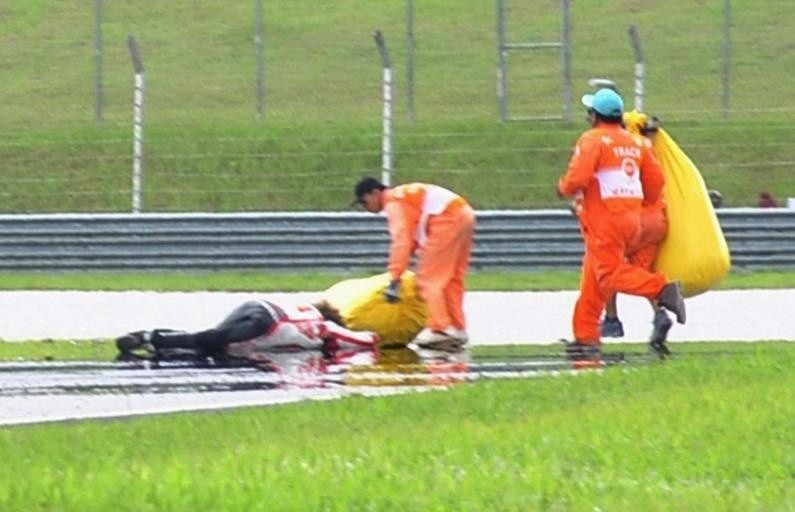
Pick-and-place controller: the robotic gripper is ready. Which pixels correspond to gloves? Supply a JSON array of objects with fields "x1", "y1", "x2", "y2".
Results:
[{"x1": 383, "y1": 278, "x2": 400, "y2": 302}]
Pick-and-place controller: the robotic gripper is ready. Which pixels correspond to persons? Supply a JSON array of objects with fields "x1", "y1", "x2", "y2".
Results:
[
  {"x1": 116, "y1": 298, "x2": 381, "y2": 359},
  {"x1": 600, "y1": 116, "x2": 672, "y2": 345},
  {"x1": 350, "y1": 178, "x2": 476, "y2": 345},
  {"x1": 116, "y1": 349, "x2": 377, "y2": 389},
  {"x1": 558, "y1": 88, "x2": 686, "y2": 353}
]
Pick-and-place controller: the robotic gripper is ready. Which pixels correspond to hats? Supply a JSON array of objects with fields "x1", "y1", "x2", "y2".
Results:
[
  {"x1": 350, "y1": 176, "x2": 381, "y2": 208},
  {"x1": 581, "y1": 87, "x2": 624, "y2": 118}
]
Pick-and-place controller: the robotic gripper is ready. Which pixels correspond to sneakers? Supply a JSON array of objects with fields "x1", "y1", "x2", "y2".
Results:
[
  {"x1": 649, "y1": 308, "x2": 674, "y2": 345},
  {"x1": 656, "y1": 279, "x2": 686, "y2": 324},
  {"x1": 598, "y1": 314, "x2": 624, "y2": 338},
  {"x1": 114, "y1": 328, "x2": 148, "y2": 354},
  {"x1": 564, "y1": 341, "x2": 601, "y2": 354}
]
